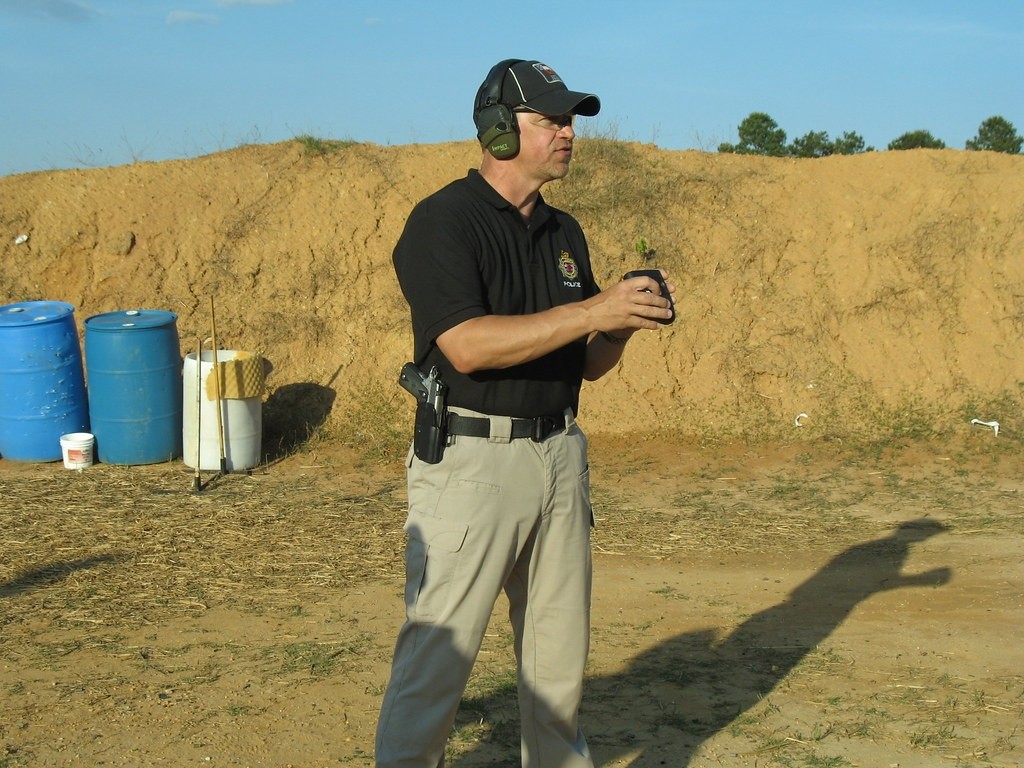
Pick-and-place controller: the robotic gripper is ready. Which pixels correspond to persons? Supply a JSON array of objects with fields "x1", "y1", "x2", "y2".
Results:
[{"x1": 372, "y1": 58, "x2": 677, "y2": 768}]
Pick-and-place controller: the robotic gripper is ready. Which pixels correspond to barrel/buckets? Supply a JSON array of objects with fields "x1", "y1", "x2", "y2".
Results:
[
  {"x1": 59, "y1": 432, "x2": 95, "y2": 469},
  {"x1": 0, "y1": 300, "x2": 89, "y2": 463},
  {"x1": 182, "y1": 350, "x2": 264, "y2": 471},
  {"x1": 83, "y1": 309, "x2": 183, "y2": 465}
]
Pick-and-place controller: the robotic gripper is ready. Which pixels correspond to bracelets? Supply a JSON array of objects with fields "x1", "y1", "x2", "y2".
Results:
[{"x1": 601, "y1": 331, "x2": 628, "y2": 344}]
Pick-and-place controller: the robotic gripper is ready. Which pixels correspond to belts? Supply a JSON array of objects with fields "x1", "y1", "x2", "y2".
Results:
[{"x1": 446, "y1": 416, "x2": 566, "y2": 442}]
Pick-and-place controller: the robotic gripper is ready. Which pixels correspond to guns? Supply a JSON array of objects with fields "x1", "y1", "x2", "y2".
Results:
[{"x1": 397, "y1": 361, "x2": 450, "y2": 465}]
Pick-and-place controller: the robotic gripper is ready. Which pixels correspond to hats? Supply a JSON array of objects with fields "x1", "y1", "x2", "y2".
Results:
[{"x1": 473, "y1": 60, "x2": 600, "y2": 125}]
